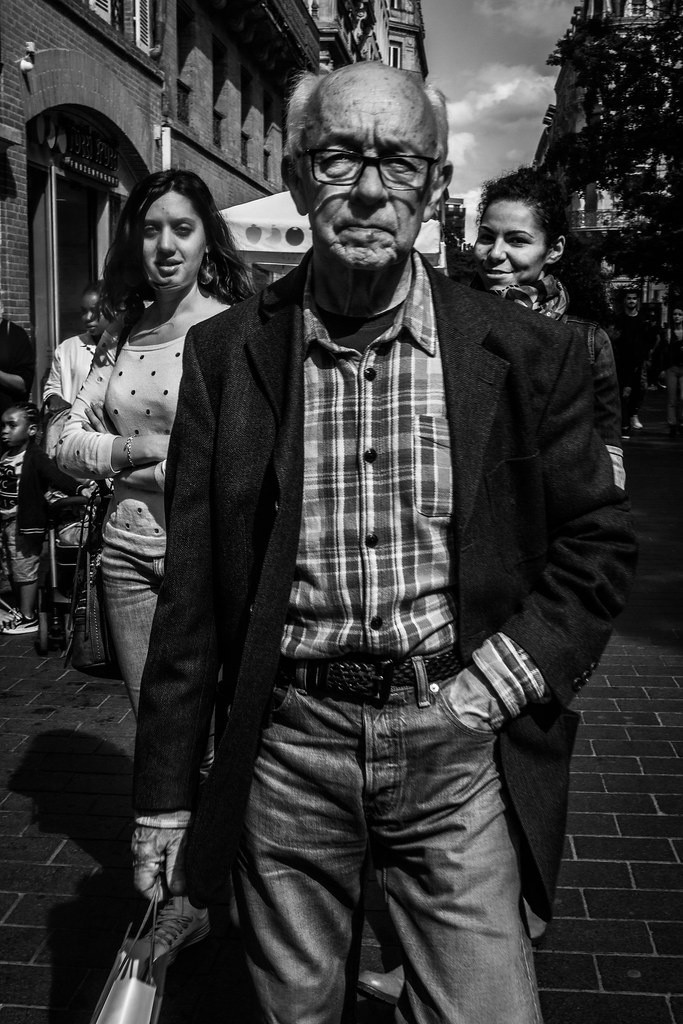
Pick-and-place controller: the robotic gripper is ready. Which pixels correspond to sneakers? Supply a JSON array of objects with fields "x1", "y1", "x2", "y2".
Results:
[
  {"x1": 136, "y1": 893, "x2": 212, "y2": 969},
  {"x1": 0, "y1": 607, "x2": 39, "y2": 633}
]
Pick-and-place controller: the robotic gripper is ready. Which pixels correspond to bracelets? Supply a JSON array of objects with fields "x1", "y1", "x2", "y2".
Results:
[{"x1": 124, "y1": 435, "x2": 136, "y2": 467}]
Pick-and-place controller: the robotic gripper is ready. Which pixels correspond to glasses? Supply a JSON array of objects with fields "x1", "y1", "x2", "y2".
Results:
[{"x1": 298, "y1": 145, "x2": 437, "y2": 191}]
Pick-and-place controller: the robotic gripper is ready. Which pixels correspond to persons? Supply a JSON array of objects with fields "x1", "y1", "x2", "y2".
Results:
[
  {"x1": 56, "y1": 172, "x2": 255, "y2": 975},
  {"x1": 131, "y1": 62, "x2": 636, "y2": 1024},
  {"x1": 0, "y1": 402, "x2": 93, "y2": 635},
  {"x1": 660, "y1": 308, "x2": 683, "y2": 434},
  {"x1": 615, "y1": 290, "x2": 653, "y2": 436},
  {"x1": 0, "y1": 300, "x2": 36, "y2": 409},
  {"x1": 468, "y1": 166, "x2": 624, "y2": 489},
  {"x1": 43, "y1": 279, "x2": 109, "y2": 415}
]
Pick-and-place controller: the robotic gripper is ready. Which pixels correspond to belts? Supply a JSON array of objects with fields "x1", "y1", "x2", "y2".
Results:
[{"x1": 278, "y1": 649, "x2": 464, "y2": 709}]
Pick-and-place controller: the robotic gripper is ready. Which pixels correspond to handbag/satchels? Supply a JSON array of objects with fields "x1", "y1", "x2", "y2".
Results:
[
  {"x1": 69, "y1": 550, "x2": 117, "y2": 678},
  {"x1": 87, "y1": 876, "x2": 168, "y2": 1024}
]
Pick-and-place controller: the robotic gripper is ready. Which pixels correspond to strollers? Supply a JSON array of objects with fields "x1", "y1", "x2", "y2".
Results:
[{"x1": 33, "y1": 494, "x2": 107, "y2": 655}]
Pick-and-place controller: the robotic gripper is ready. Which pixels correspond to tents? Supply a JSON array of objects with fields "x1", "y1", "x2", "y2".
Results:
[{"x1": 218, "y1": 191, "x2": 448, "y2": 273}]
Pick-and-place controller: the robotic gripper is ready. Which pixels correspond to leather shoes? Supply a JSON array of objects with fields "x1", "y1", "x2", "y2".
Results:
[{"x1": 355, "y1": 962, "x2": 406, "y2": 1003}]
